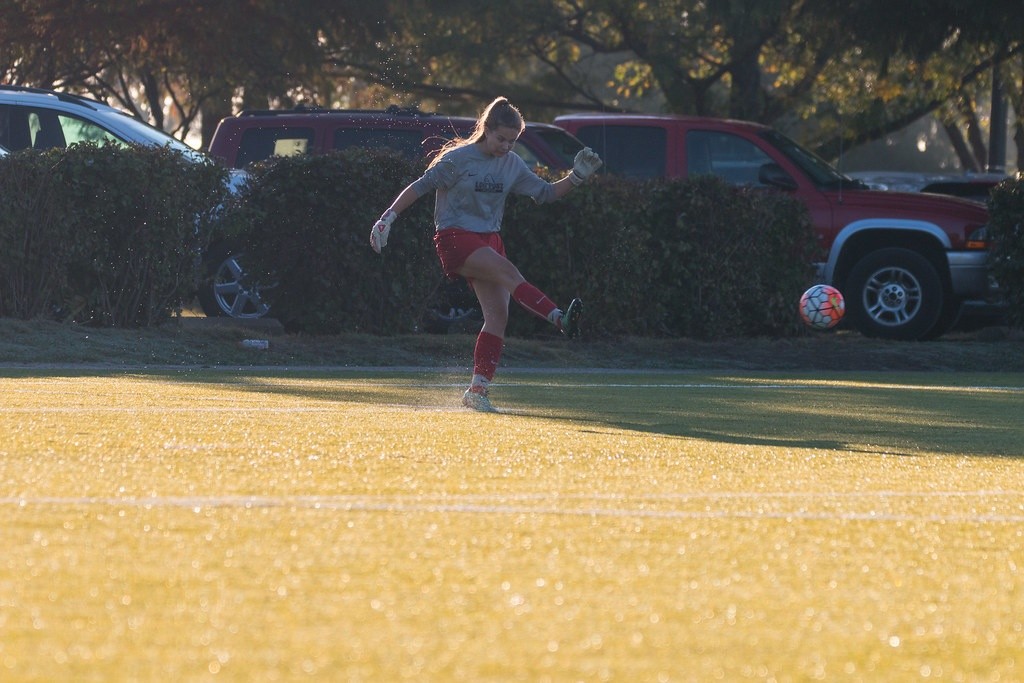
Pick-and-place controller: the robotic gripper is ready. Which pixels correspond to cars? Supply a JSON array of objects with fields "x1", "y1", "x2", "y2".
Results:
[{"x1": 849, "y1": 165, "x2": 1020, "y2": 205}]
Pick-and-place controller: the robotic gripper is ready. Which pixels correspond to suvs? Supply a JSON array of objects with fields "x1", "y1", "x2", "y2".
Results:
[
  {"x1": 204, "y1": 100, "x2": 606, "y2": 191},
  {"x1": 548, "y1": 109, "x2": 1004, "y2": 339},
  {"x1": 0, "y1": 79, "x2": 281, "y2": 322}
]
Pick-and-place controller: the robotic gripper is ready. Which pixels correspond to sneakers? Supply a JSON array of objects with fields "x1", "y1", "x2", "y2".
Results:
[
  {"x1": 461, "y1": 388, "x2": 498, "y2": 413},
  {"x1": 560, "y1": 297, "x2": 583, "y2": 339}
]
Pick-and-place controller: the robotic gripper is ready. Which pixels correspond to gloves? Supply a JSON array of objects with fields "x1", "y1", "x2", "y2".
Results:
[
  {"x1": 568, "y1": 146, "x2": 602, "y2": 186},
  {"x1": 371, "y1": 209, "x2": 397, "y2": 253}
]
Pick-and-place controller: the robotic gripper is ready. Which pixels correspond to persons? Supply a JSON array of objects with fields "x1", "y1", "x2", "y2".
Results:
[{"x1": 371, "y1": 94, "x2": 602, "y2": 411}]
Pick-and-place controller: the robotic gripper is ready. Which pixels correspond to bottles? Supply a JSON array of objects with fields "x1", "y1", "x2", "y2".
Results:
[{"x1": 241, "y1": 339, "x2": 268, "y2": 349}]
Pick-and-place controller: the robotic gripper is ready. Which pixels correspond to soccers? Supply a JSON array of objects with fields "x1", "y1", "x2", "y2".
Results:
[{"x1": 799, "y1": 284, "x2": 845, "y2": 329}]
